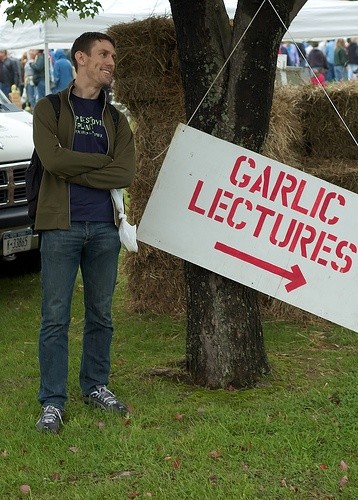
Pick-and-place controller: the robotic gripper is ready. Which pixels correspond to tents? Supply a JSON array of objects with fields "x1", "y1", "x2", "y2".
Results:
[
  {"x1": 224, "y1": 0, "x2": 358, "y2": 40},
  {"x1": 0, "y1": 0, "x2": 172, "y2": 96}
]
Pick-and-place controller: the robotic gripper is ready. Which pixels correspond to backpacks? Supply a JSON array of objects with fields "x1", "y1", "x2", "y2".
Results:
[{"x1": 26, "y1": 94, "x2": 119, "y2": 220}]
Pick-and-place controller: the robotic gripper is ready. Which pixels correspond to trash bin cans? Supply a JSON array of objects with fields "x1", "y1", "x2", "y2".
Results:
[{"x1": 283, "y1": 67, "x2": 311, "y2": 86}]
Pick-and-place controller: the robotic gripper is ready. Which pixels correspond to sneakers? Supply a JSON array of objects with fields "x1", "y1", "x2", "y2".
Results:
[
  {"x1": 84, "y1": 385, "x2": 129, "y2": 417},
  {"x1": 36, "y1": 405, "x2": 65, "y2": 436}
]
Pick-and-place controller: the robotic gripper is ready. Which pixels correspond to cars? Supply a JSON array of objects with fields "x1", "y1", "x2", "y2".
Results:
[{"x1": 0, "y1": 89, "x2": 42, "y2": 273}]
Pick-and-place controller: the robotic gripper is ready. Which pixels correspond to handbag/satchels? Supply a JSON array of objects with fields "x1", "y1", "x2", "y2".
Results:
[{"x1": 67, "y1": 61, "x2": 77, "y2": 79}]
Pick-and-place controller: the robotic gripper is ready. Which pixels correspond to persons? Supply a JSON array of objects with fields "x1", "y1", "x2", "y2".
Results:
[
  {"x1": 0, "y1": 48, "x2": 24, "y2": 98},
  {"x1": 20, "y1": 49, "x2": 55, "y2": 113},
  {"x1": 33, "y1": 32, "x2": 136, "y2": 435},
  {"x1": 53, "y1": 49, "x2": 74, "y2": 91},
  {"x1": 278, "y1": 41, "x2": 308, "y2": 68},
  {"x1": 306, "y1": 41, "x2": 329, "y2": 84},
  {"x1": 321, "y1": 36, "x2": 358, "y2": 82}
]
